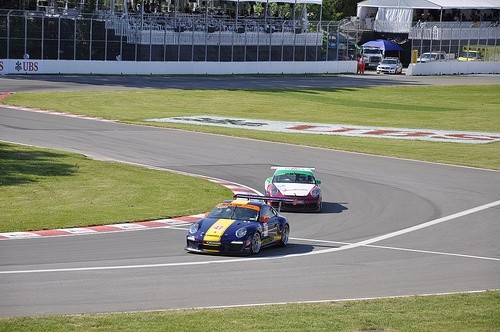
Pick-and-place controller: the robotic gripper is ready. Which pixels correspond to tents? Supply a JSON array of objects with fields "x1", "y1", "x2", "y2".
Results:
[{"x1": 361, "y1": 39, "x2": 404, "y2": 59}]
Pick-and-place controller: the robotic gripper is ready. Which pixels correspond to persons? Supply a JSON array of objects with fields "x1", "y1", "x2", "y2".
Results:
[
  {"x1": 208, "y1": 9, "x2": 236, "y2": 19},
  {"x1": 127, "y1": 1, "x2": 162, "y2": 15},
  {"x1": 365, "y1": 12, "x2": 376, "y2": 18},
  {"x1": 239, "y1": 5, "x2": 254, "y2": 17},
  {"x1": 416, "y1": 10, "x2": 499, "y2": 22},
  {"x1": 258, "y1": 6, "x2": 291, "y2": 20},
  {"x1": 184, "y1": 3, "x2": 201, "y2": 14},
  {"x1": 356, "y1": 54, "x2": 366, "y2": 74}
]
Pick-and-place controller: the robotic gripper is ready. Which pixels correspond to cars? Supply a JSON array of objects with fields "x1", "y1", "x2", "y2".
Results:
[
  {"x1": 458, "y1": 50, "x2": 482, "y2": 62},
  {"x1": 376, "y1": 56, "x2": 403, "y2": 75}
]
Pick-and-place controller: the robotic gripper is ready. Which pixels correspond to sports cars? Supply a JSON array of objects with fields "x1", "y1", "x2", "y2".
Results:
[
  {"x1": 184, "y1": 193, "x2": 289, "y2": 256},
  {"x1": 264, "y1": 165, "x2": 323, "y2": 213}
]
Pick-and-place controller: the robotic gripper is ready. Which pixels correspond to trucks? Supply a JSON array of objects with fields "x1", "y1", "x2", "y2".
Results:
[{"x1": 361, "y1": 47, "x2": 384, "y2": 68}]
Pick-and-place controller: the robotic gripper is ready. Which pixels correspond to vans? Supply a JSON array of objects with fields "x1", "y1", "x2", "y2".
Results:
[{"x1": 417, "y1": 52, "x2": 448, "y2": 63}]
